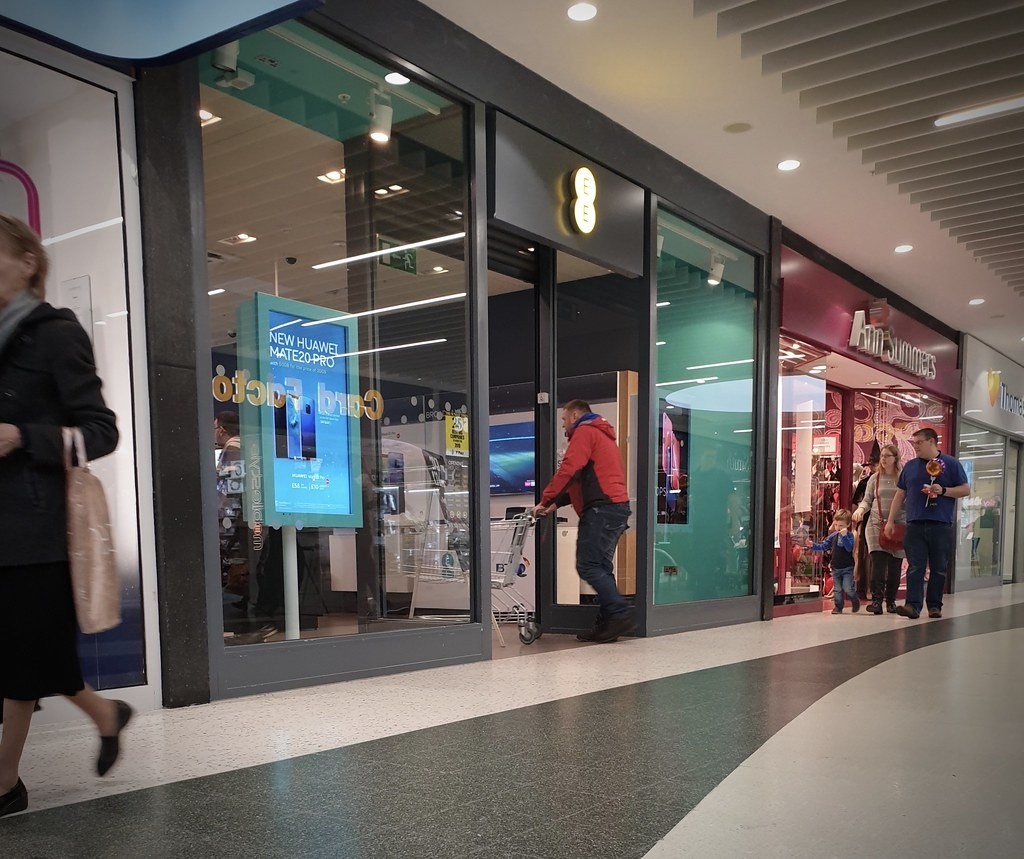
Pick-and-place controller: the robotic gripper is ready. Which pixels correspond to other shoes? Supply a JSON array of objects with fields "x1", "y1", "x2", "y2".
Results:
[
  {"x1": 897, "y1": 604, "x2": 919, "y2": 619},
  {"x1": 866, "y1": 603, "x2": 883, "y2": 614},
  {"x1": 832, "y1": 606, "x2": 842, "y2": 614},
  {"x1": 886, "y1": 603, "x2": 896, "y2": 613},
  {"x1": 852, "y1": 602, "x2": 860, "y2": 612},
  {"x1": 929, "y1": 608, "x2": 942, "y2": 617},
  {"x1": 96, "y1": 699, "x2": 131, "y2": 776},
  {"x1": 0, "y1": 776, "x2": 28, "y2": 817}
]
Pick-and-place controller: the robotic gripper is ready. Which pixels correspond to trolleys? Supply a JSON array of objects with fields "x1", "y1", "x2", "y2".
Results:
[{"x1": 396, "y1": 508, "x2": 544, "y2": 645}]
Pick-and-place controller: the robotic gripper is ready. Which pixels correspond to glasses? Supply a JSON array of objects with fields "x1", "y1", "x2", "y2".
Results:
[
  {"x1": 913, "y1": 439, "x2": 928, "y2": 445},
  {"x1": 879, "y1": 454, "x2": 896, "y2": 458}
]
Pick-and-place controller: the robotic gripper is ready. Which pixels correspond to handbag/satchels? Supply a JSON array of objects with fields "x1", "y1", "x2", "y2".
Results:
[
  {"x1": 878, "y1": 522, "x2": 905, "y2": 550},
  {"x1": 62, "y1": 426, "x2": 122, "y2": 634}
]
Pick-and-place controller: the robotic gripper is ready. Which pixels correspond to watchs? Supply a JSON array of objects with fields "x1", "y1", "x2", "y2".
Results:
[{"x1": 938, "y1": 486, "x2": 946, "y2": 496}]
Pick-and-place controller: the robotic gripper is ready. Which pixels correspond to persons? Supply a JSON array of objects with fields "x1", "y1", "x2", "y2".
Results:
[
  {"x1": 883, "y1": 427, "x2": 970, "y2": 619},
  {"x1": 852, "y1": 445, "x2": 907, "y2": 614},
  {"x1": 805, "y1": 509, "x2": 861, "y2": 614},
  {"x1": 530, "y1": 399, "x2": 641, "y2": 643},
  {"x1": 0, "y1": 215, "x2": 132, "y2": 816},
  {"x1": 215, "y1": 411, "x2": 255, "y2": 638},
  {"x1": 689, "y1": 444, "x2": 738, "y2": 601}
]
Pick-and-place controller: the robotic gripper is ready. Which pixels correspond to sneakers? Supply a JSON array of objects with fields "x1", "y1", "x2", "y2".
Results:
[
  {"x1": 577, "y1": 614, "x2": 604, "y2": 641},
  {"x1": 593, "y1": 618, "x2": 638, "y2": 642}
]
